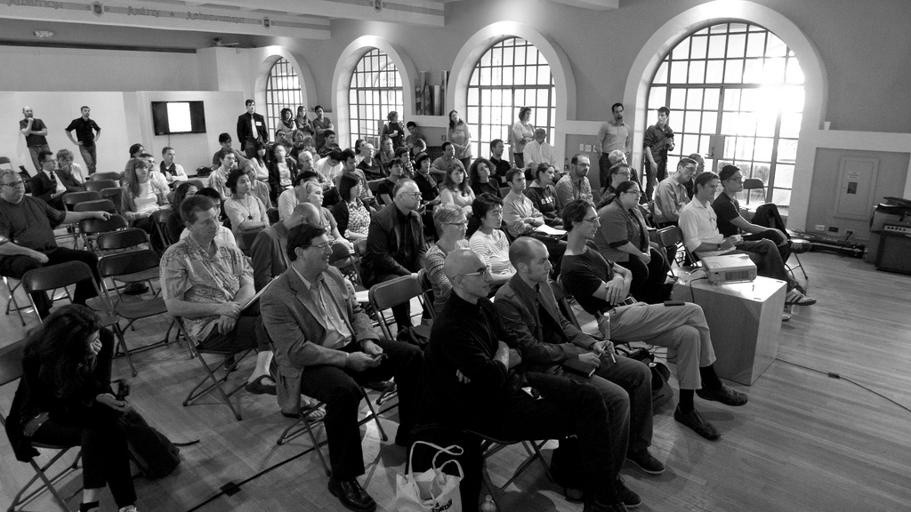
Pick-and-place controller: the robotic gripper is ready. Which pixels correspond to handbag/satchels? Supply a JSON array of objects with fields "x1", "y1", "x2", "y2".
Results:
[
  {"x1": 620, "y1": 345, "x2": 673, "y2": 410},
  {"x1": 113, "y1": 378, "x2": 180, "y2": 476},
  {"x1": 392, "y1": 442, "x2": 465, "y2": 511}
]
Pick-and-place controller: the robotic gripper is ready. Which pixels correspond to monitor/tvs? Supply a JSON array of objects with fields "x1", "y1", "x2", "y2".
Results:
[{"x1": 151, "y1": 100, "x2": 206, "y2": 136}]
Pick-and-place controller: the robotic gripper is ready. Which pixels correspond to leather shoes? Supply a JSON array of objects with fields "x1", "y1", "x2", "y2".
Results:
[{"x1": 328, "y1": 475, "x2": 378, "y2": 512}]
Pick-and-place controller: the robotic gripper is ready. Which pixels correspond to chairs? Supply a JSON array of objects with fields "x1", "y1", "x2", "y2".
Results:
[
  {"x1": 1, "y1": 336, "x2": 85, "y2": 512},
  {"x1": 1, "y1": 156, "x2": 177, "y2": 331},
  {"x1": 21, "y1": 261, "x2": 137, "y2": 382},
  {"x1": 168, "y1": 177, "x2": 808, "y2": 507},
  {"x1": 97, "y1": 249, "x2": 194, "y2": 377}
]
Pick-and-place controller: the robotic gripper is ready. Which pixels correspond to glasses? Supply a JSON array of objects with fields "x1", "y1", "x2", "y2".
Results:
[{"x1": 465, "y1": 264, "x2": 490, "y2": 278}]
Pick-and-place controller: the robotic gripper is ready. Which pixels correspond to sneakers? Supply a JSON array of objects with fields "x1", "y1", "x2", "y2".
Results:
[
  {"x1": 785, "y1": 288, "x2": 816, "y2": 305},
  {"x1": 627, "y1": 445, "x2": 665, "y2": 475},
  {"x1": 695, "y1": 382, "x2": 748, "y2": 406},
  {"x1": 673, "y1": 403, "x2": 721, "y2": 441},
  {"x1": 612, "y1": 475, "x2": 641, "y2": 506}
]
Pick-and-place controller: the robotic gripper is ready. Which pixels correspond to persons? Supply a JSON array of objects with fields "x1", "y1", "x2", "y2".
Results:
[
  {"x1": 30, "y1": 152, "x2": 88, "y2": 211},
  {"x1": 1, "y1": 169, "x2": 112, "y2": 319},
  {"x1": 121, "y1": 100, "x2": 814, "y2": 509},
  {"x1": 20, "y1": 105, "x2": 50, "y2": 172},
  {"x1": 65, "y1": 106, "x2": 101, "y2": 175},
  {"x1": 5, "y1": 303, "x2": 138, "y2": 511},
  {"x1": 57, "y1": 149, "x2": 86, "y2": 183},
  {"x1": 1, "y1": 158, "x2": 32, "y2": 193}
]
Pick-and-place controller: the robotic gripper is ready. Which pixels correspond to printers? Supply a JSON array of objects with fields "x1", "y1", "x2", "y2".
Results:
[{"x1": 869, "y1": 195, "x2": 911, "y2": 235}]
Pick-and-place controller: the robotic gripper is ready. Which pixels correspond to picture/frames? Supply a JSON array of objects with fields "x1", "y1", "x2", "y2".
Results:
[{"x1": 830, "y1": 154, "x2": 879, "y2": 224}]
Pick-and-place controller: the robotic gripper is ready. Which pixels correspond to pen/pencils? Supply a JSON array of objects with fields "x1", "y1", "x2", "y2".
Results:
[{"x1": 610, "y1": 352, "x2": 617, "y2": 363}]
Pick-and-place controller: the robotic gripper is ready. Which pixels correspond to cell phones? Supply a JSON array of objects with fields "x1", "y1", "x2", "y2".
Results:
[{"x1": 663, "y1": 299, "x2": 685, "y2": 306}]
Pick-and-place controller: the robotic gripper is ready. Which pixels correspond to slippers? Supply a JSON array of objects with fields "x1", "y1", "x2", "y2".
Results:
[
  {"x1": 281, "y1": 401, "x2": 325, "y2": 421},
  {"x1": 245, "y1": 374, "x2": 278, "y2": 395}
]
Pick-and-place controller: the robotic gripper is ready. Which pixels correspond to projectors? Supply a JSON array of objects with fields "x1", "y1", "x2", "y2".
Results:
[{"x1": 701, "y1": 252, "x2": 759, "y2": 284}]
